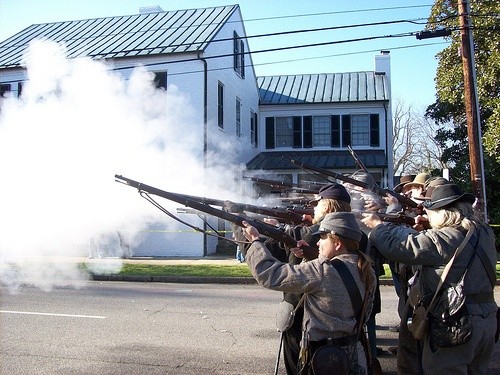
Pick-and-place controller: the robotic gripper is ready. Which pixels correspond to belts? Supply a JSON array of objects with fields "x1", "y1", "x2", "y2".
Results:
[
  {"x1": 464, "y1": 291, "x2": 495, "y2": 306},
  {"x1": 308, "y1": 335, "x2": 360, "y2": 351}
]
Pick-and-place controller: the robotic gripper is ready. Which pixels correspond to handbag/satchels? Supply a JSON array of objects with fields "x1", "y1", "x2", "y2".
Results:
[
  {"x1": 429, "y1": 280, "x2": 472, "y2": 346},
  {"x1": 409, "y1": 306, "x2": 429, "y2": 340},
  {"x1": 300, "y1": 346, "x2": 349, "y2": 375},
  {"x1": 272, "y1": 301, "x2": 294, "y2": 332}
]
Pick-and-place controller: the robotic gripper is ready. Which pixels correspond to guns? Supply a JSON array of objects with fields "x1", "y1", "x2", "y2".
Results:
[
  {"x1": 113, "y1": 174, "x2": 320, "y2": 262},
  {"x1": 233, "y1": 139, "x2": 432, "y2": 230},
  {"x1": 172, "y1": 192, "x2": 315, "y2": 227}
]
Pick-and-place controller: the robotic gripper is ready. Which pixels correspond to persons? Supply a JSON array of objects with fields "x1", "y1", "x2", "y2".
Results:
[
  {"x1": 261, "y1": 168, "x2": 452, "y2": 375},
  {"x1": 220, "y1": 175, "x2": 356, "y2": 375},
  {"x1": 362, "y1": 183, "x2": 499, "y2": 375},
  {"x1": 239, "y1": 211, "x2": 377, "y2": 375}
]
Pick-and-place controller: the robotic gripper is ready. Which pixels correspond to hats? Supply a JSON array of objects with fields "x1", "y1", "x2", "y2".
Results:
[
  {"x1": 414, "y1": 177, "x2": 448, "y2": 200},
  {"x1": 309, "y1": 183, "x2": 351, "y2": 203},
  {"x1": 348, "y1": 169, "x2": 375, "y2": 185},
  {"x1": 422, "y1": 184, "x2": 476, "y2": 210},
  {"x1": 421, "y1": 177, "x2": 436, "y2": 195},
  {"x1": 394, "y1": 175, "x2": 416, "y2": 192},
  {"x1": 311, "y1": 212, "x2": 363, "y2": 241},
  {"x1": 403, "y1": 173, "x2": 431, "y2": 187}
]
